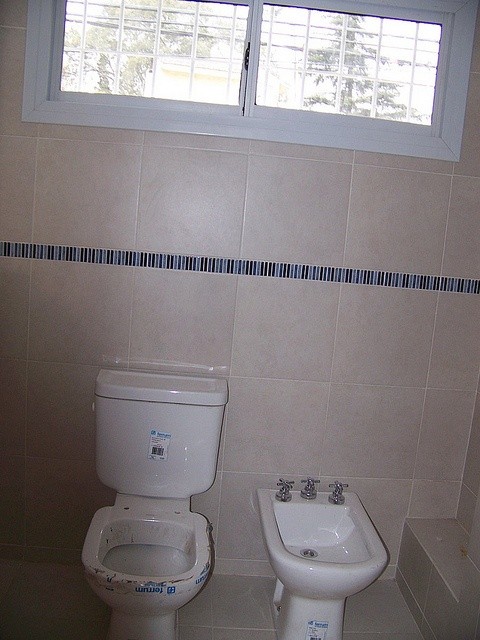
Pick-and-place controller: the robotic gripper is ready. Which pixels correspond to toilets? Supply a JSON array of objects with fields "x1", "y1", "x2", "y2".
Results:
[{"x1": 80, "y1": 368, "x2": 230, "y2": 640}]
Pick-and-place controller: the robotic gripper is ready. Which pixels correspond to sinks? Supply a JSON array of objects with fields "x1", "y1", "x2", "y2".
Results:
[{"x1": 254, "y1": 486, "x2": 389, "y2": 640}]
